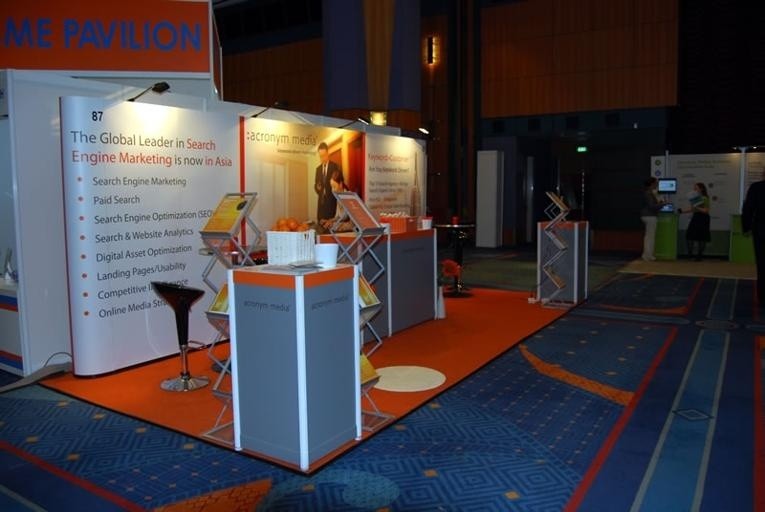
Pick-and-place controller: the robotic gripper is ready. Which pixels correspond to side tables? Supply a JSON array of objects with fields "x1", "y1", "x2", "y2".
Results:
[{"x1": 434, "y1": 223, "x2": 475, "y2": 299}]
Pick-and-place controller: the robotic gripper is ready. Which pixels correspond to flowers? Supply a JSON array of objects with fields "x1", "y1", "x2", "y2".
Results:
[{"x1": 438, "y1": 257, "x2": 462, "y2": 285}]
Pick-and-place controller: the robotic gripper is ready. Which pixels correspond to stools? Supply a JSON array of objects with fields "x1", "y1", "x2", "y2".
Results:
[{"x1": 152, "y1": 280, "x2": 212, "y2": 392}]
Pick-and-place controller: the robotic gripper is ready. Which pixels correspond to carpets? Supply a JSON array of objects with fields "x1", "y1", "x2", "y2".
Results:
[
  {"x1": 617, "y1": 250, "x2": 758, "y2": 280},
  {"x1": 38, "y1": 285, "x2": 574, "y2": 477}
]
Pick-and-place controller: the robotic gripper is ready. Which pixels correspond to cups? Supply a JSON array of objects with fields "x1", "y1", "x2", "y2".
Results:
[
  {"x1": 313, "y1": 243, "x2": 338, "y2": 267},
  {"x1": 452, "y1": 215, "x2": 459, "y2": 224},
  {"x1": 422, "y1": 220, "x2": 432, "y2": 229}
]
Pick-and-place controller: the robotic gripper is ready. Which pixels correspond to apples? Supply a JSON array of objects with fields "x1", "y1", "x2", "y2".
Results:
[{"x1": 272, "y1": 217, "x2": 310, "y2": 232}]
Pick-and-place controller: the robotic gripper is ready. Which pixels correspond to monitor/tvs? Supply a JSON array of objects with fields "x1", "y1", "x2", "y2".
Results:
[{"x1": 658, "y1": 177, "x2": 677, "y2": 195}]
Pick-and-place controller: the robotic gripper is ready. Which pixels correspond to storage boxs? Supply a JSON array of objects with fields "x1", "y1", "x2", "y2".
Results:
[{"x1": 381, "y1": 214, "x2": 418, "y2": 233}]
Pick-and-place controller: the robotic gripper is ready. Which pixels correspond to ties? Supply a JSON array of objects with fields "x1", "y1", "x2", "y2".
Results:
[{"x1": 323, "y1": 164, "x2": 326, "y2": 189}]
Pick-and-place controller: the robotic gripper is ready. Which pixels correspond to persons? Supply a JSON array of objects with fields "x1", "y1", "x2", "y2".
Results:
[
  {"x1": 318, "y1": 171, "x2": 355, "y2": 232},
  {"x1": 639, "y1": 177, "x2": 672, "y2": 262},
  {"x1": 677, "y1": 183, "x2": 711, "y2": 261},
  {"x1": 741, "y1": 167, "x2": 765, "y2": 318},
  {"x1": 314, "y1": 143, "x2": 340, "y2": 231}
]
